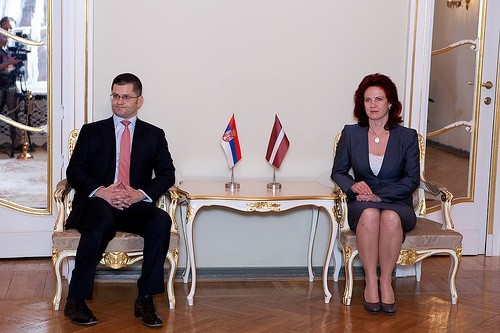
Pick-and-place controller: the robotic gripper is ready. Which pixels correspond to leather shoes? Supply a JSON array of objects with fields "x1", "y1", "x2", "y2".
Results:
[
  {"x1": 64, "y1": 300, "x2": 98, "y2": 325},
  {"x1": 133, "y1": 298, "x2": 164, "y2": 327}
]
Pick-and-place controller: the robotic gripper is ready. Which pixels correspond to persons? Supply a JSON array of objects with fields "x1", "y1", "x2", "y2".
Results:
[
  {"x1": 330, "y1": 72, "x2": 421, "y2": 316},
  {"x1": 0, "y1": 17, "x2": 24, "y2": 152},
  {"x1": 63, "y1": 73, "x2": 176, "y2": 328}
]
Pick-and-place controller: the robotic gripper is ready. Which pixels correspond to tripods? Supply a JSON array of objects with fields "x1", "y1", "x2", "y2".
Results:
[{"x1": 0, "y1": 68, "x2": 34, "y2": 158}]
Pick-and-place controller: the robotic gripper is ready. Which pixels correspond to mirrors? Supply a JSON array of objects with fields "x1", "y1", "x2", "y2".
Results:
[
  {"x1": 0, "y1": 1, "x2": 52, "y2": 216},
  {"x1": 422, "y1": 0, "x2": 486, "y2": 215}
]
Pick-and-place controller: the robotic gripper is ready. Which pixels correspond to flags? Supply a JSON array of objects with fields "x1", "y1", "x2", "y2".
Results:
[
  {"x1": 265, "y1": 114, "x2": 291, "y2": 169},
  {"x1": 220, "y1": 114, "x2": 243, "y2": 170}
]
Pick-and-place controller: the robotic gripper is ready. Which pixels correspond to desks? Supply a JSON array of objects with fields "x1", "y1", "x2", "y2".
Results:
[{"x1": 174, "y1": 180, "x2": 342, "y2": 306}]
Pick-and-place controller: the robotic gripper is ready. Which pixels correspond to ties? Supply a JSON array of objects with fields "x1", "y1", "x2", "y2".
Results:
[{"x1": 118, "y1": 120, "x2": 131, "y2": 189}]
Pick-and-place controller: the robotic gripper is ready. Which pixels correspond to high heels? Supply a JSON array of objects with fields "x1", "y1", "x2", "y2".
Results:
[
  {"x1": 379, "y1": 288, "x2": 396, "y2": 315},
  {"x1": 362, "y1": 288, "x2": 381, "y2": 314}
]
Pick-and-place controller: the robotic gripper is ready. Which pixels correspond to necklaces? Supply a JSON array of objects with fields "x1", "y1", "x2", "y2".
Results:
[{"x1": 369, "y1": 125, "x2": 386, "y2": 144}]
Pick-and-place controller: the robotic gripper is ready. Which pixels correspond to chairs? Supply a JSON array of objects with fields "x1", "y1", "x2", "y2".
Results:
[
  {"x1": 52, "y1": 129, "x2": 179, "y2": 311},
  {"x1": 334, "y1": 134, "x2": 463, "y2": 306}
]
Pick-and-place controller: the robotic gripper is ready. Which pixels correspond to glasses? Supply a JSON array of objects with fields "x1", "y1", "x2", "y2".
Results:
[{"x1": 110, "y1": 93, "x2": 138, "y2": 100}]
